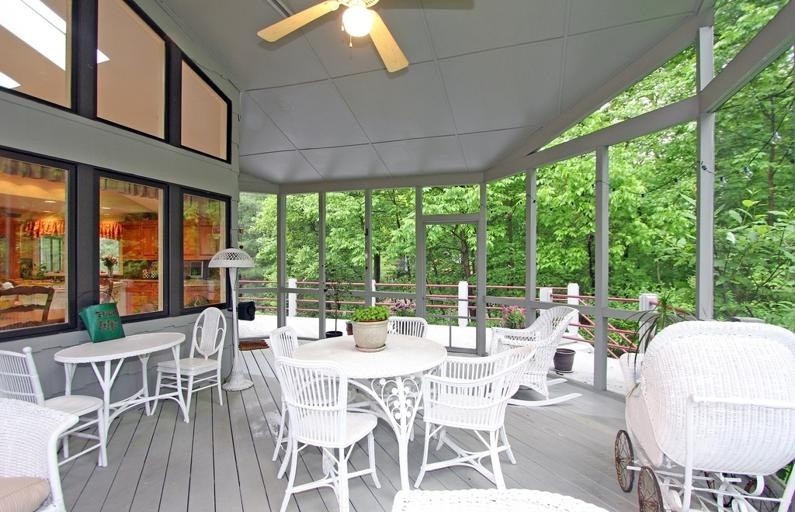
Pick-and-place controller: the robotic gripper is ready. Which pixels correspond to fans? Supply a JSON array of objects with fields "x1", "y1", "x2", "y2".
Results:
[{"x1": 255, "y1": 0, "x2": 411, "y2": 75}]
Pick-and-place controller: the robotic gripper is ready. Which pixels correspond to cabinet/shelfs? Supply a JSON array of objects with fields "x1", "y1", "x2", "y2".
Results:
[
  {"x1": 183, "y1": 286, "x2": 220, "y2": 307},
  {"x1": 120, "y1": 222, "x2": 157, "y2": 259},
  {"x1": 183, "y1": 219, "x2": 217, "y2": 261},
  {"x1": 125, "y1": 282, "x2": 158, "y2": 314}
]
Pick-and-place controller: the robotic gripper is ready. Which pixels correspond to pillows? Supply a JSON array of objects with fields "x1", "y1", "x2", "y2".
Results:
[{"x1": 0, "y1": 474, "x2": 52, "y2": 512}]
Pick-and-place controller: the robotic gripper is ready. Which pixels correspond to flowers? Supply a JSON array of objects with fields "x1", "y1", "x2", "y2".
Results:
[{"x1": 100, "y1": 256, "x2": 119, "y2": 275}]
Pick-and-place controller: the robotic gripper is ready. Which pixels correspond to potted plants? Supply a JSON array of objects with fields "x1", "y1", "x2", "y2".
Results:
[
  {"x1": 323, "y1": 275, "x2": 352, "y2": 340},
  {"x1": 349, "y1": 307, "x2": 390, "y2": 352}
]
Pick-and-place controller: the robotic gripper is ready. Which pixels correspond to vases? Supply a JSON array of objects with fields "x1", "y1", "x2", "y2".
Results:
[{"x1": 107, "y1": 266, "x2": 113, "y2": 278}]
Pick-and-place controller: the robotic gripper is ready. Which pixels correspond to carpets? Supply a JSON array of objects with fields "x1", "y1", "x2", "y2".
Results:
[{"x1": 240, "y1": 336, "x2": 268, "y2": 350}]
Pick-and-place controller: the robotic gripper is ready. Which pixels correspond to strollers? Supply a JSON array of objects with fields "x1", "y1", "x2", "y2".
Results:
[{"x1": 612, "y1": 291, "x2": 795, "y2": 512}]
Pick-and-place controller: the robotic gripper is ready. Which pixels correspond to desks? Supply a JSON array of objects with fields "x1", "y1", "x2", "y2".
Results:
[
  {"x1": 53, "y1": 331, "x2": 190, "y2": 466},
  {"x1": 293, "y1": 333, "x2": 448, "y2": 490}
]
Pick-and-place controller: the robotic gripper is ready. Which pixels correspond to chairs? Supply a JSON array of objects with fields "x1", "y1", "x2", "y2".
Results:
[
  {"x1": 0, "y1": 397, "x2": 80, "y2": 512},
  {"x1": 267, "y1": 326, "x2": 336, "y2": 480},
  {"x1": 413, "y1": 344, "x2": 539, "y2": 489},
  {"x1": 387, "y1": 316, "x2": 427, "y2": 337},
  {"x1": 491, "y1": 306, "x2": 583, "y2": 407},
  {"x1": 275, "y1": 355, "x2": 381, "y2": 512},
  {"x1": 150, "y1": 307, "x2": 227, "y2": 424},
  {"x1": 0, "y1": 346, "x2": 108, "y2": 467}
]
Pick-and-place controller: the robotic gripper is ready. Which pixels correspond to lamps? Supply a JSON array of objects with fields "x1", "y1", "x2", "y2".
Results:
[{"x1": 341, "y1": 1, "x2": 375, "y2": 38}]
